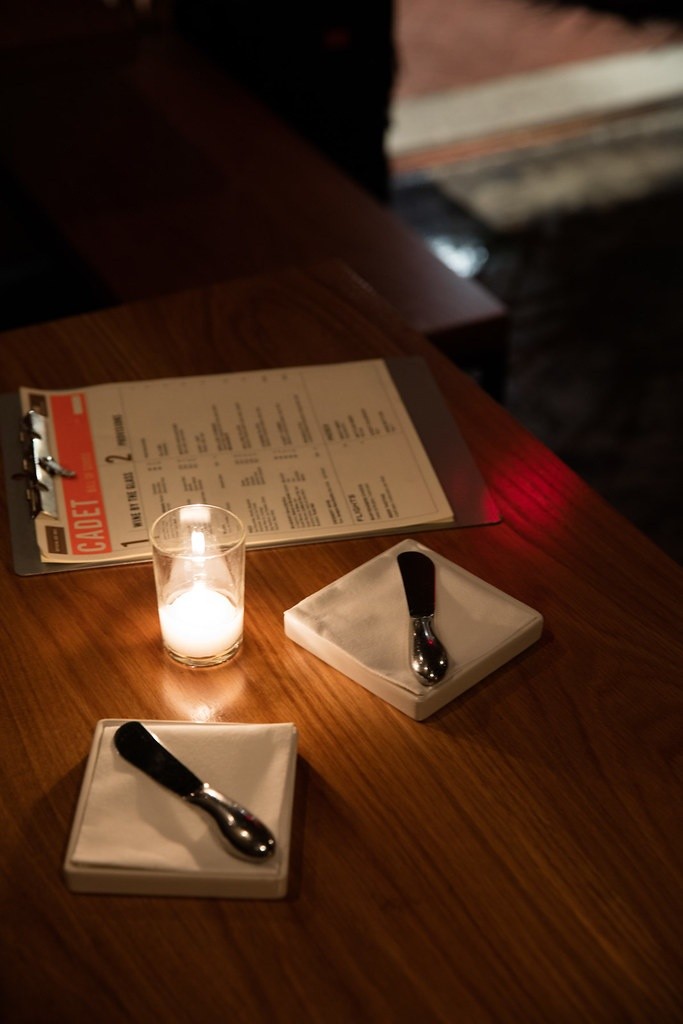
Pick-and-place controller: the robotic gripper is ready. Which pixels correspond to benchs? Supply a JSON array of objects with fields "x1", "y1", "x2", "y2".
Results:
[{"x1": 0, "y1": 1, "x2": 513, "y2": 409}]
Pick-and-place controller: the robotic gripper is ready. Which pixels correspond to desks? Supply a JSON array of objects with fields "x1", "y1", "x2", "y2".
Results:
[{"x1": 0, "y1": 258, "x2": 681, "y2": 1024}]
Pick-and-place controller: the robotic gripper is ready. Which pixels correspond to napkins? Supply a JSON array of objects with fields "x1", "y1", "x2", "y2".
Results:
[
  {"x1": 283, "y1": 538, "x2": 537, "y2": 696},
  {"x1": 71, "y1": 722, "x2": 296, "y2": 877}
]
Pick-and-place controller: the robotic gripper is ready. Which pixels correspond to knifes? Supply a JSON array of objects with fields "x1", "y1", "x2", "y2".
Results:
[
  {"x1": 110, "y1": 720, "x2": 277, "y2": 861},
  {"x1": 397, "y1": 552, "x2": 451, "y2": 686}
]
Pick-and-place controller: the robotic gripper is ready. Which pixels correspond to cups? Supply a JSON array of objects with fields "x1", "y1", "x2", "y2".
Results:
[{"x1": 147, "y1": 503, "x2": 246, "y2": 671}]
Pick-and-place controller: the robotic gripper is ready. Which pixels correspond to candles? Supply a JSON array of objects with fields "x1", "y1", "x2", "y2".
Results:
[{"x1": 158, "y1": 586, "x2": 243, "y2": 657}]
